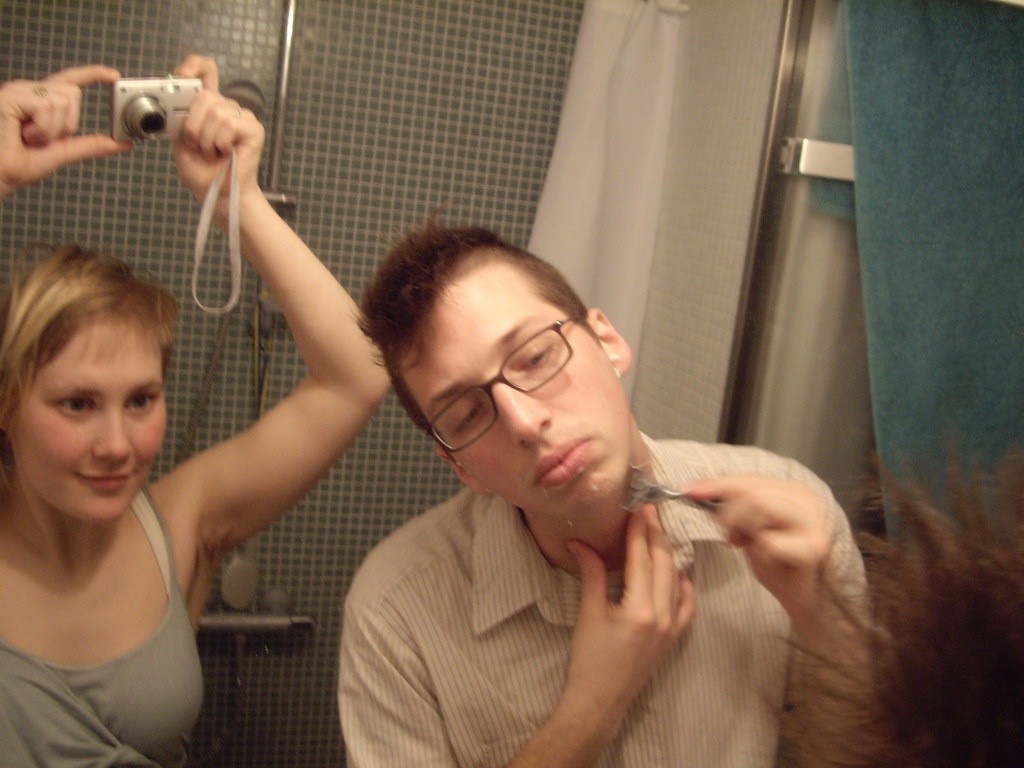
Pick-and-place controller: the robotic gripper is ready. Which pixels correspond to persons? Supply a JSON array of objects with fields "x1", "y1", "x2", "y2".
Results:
[
  {"x1": 338, "y1": 227, "x2": 891, "y2": 768},
  {"x1": 0, "y1": 56, "x2": 391, "y2": 768},
  {"x1": 760, "y1": 447, "x2": 1024, "y2": 768}
]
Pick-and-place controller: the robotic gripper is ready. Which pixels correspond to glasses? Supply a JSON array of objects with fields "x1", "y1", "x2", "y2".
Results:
[{"x1": 428, "y1": 315, "x2": 585, "y2": 453}]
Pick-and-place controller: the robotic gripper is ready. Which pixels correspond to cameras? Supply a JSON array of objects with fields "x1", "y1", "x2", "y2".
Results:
[{"x1": 110, "y1": 76, "x2": 203, "y2": 141}]
